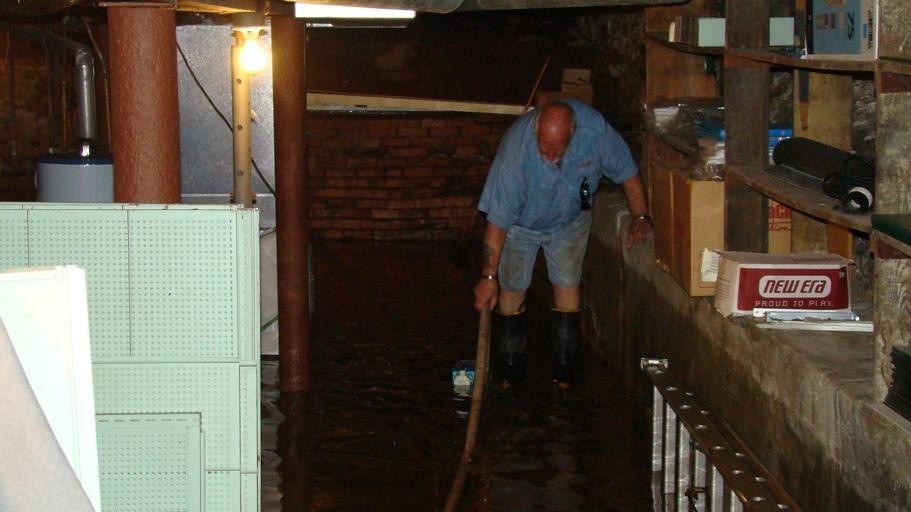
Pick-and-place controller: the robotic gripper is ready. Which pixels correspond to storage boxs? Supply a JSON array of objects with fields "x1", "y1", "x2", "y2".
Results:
[
  {"x1": 655, "y1": 161, "x2": 697, "y2": 282},
  {"x1": 674, "y1": 171, "x2": 795, "y2": 298}
]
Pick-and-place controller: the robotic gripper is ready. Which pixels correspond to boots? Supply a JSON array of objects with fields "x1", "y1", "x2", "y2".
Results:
[
  {"x1": 551, "y1": 309, "x2": 584, "y2": 392},
  {"x1": 494, "y1": 313, "x2": 520, "y2": 393}
]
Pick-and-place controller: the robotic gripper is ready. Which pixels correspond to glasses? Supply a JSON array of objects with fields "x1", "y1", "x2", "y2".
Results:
[{"x1": 579, "y1": 179, "x2": 590, "y2": 211}]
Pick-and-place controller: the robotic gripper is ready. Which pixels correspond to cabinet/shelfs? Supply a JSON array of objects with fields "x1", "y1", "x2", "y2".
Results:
[{"x1": 645, "y1": 0, "x2": 911, "y2": 430}]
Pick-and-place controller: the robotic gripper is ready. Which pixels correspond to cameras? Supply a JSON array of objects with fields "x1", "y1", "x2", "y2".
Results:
[{"x1": 822, "y1": 157, "x2": 874, "y2": 215}]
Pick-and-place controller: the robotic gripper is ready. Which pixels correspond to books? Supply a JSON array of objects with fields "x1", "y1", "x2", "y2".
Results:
[{"x1": 697, "y1": 128, "x2": 794, "y2": 166}]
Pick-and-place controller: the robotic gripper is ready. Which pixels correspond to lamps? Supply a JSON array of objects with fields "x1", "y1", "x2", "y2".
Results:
[
  {"x1": 231, "y1": 14, "x2": 273, "y2": 75},
  {"x1": 293, "y1": 1, "x2": 417, "y2": 21}
]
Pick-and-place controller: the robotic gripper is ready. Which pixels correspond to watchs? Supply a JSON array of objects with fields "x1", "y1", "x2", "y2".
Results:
[
  {"x1": 480, "y1": 275, "x2": 499, "y2": 281},
  {"x1": 633, "y1": 215, "x2": 653, "y2": 220}
]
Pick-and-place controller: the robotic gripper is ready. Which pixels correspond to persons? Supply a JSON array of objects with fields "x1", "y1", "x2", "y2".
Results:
[{"x1": 474, "y1": 102, "x2": 651, "y2": 383}]
